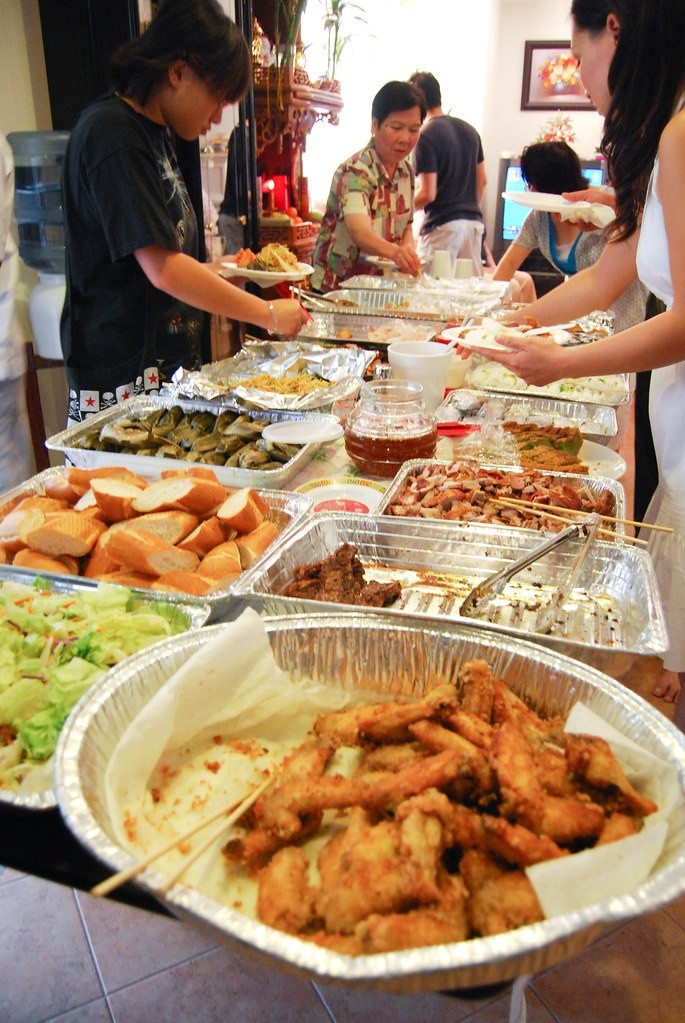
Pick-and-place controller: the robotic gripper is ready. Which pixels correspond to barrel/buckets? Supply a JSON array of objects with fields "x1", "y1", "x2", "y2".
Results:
[
  {"x1": 386, "y1": 339, "x2": 454, "y2": 415},
  {"x1": 5, "y1": 128, "x2": 79, "y2": 276}
]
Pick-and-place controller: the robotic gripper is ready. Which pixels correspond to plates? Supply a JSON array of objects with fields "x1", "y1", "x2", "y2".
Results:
[
  {"x1": 293, "y1": 477, "x2": 386, "y2": 523},
  {"x1": 222, "y1": 262, "x2": 314, "y2": 277},
  {"x1": 501, "y1": 188, "x2": 590, "y2": 214}
]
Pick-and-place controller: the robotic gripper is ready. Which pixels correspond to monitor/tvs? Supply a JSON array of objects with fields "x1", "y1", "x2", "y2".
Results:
[{"x1": 493, "y1": 158, "x2": 610, "y2": 274}]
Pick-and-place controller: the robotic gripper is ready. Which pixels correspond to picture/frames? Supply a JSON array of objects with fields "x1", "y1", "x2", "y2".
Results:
[{"x1": 520, "y1": 40, "x2": 598, "y2": 112}]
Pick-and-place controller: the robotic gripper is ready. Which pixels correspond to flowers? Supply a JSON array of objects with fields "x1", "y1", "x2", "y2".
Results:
[{"x1": 530, "y1": 115, "x2": 577, "y2": 146}]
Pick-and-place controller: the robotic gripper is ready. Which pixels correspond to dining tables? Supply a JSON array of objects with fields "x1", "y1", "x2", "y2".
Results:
[{"x1": 0, "y1": 301, "x2": 637, "y2": 1002}]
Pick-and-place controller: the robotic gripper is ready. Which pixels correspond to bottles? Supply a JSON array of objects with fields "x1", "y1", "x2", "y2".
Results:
[{"x1": 344, "y1": 379, "x2": 438, "y2": 476}]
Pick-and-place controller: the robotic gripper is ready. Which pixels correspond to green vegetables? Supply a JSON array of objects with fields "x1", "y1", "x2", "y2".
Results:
[{"x1": 0, "y1": 574, "x2": 197, "y2": 796}]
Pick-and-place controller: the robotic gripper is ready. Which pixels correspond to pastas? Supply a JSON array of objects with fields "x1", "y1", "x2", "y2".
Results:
[
  {"x1": 271, "y1": 250, "x2": 298, "y2": 272},
  {"x1": 224, "y1": 372, "x2": 324, "y2": 393}
]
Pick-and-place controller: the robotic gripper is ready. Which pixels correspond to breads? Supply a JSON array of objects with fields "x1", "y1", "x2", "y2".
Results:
[{"x1": 1, "y1": 465, "x2": 279, "y2": 595}]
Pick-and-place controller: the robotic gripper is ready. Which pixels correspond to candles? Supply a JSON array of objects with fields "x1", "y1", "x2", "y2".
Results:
[{"x1": 267, "y1": 180, "x2": 275, "y2": 211}]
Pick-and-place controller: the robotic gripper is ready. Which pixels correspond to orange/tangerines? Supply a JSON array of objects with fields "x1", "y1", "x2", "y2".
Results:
[{"x1": 284, "y1": 206, "x2": 302, "y2": 224}]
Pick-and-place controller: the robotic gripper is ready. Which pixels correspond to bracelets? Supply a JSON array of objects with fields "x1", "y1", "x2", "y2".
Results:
[{"x1": 266, "y1": 301, "x2": 279, "y2": 337}]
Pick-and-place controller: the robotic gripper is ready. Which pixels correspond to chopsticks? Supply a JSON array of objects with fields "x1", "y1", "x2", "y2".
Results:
[
  {"x1": 488, "y1": 496, "x2": 675, "y2": 545},
  {"x1": 290, "y1": 283, "x2": 302, "y2": 304}
]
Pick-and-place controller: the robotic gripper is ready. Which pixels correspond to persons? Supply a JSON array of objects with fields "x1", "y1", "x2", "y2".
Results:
[
  {"x1": 490, "y1": 139, "x2": 647, "y2": 335},
  {"x1": 307, "y1": 81, "x2": 427, "y2": 297},
  {"x1": 456, "y1": 0, "x2": 685, "y2": 732},
  {"x1": 0, "y1": 138, "x2": 36, "y2": 493},
  {"x1": 409, "y1": 73, "x2": 487, "y2": 280},
  {"x1": 59, "y1": 0, "x2": 309, "y2": 468},
  {"x1": 217, "y1": 125, "x2": 246, "y2": 254}
]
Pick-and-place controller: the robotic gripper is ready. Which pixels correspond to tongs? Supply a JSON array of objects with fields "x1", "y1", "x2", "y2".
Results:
[
  {"x1": 289, "y1": 285, "x2": 359, "y2": 314},
  {"x1": 458, "y1": 512, "x2": 602, "y2": 633}
]
